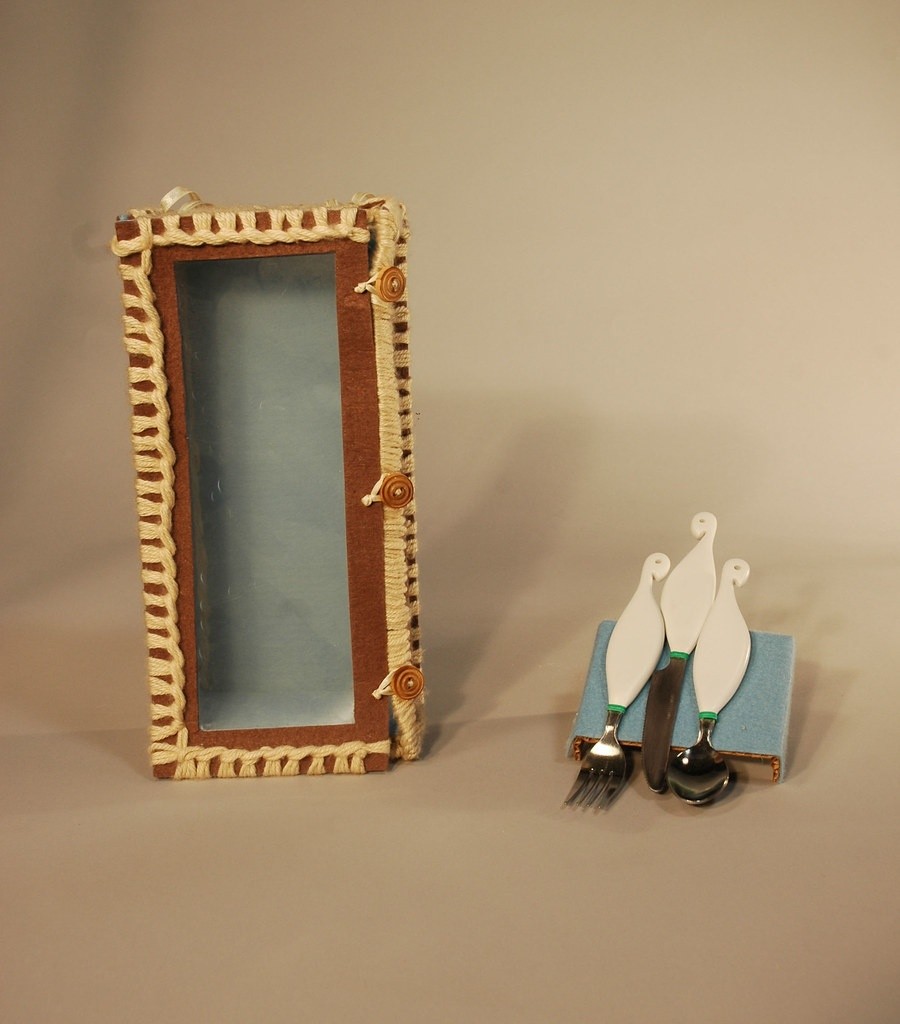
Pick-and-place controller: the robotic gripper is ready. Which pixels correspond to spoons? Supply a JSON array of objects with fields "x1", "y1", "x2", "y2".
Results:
[{"x1": 667, "y1": 558, "x2": 754, "y2": 804}]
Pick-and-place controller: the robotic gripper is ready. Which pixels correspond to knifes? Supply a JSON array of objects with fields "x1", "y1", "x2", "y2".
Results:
[{"x1": 642, "y1": 512, "x2": 717, "y2": 793}]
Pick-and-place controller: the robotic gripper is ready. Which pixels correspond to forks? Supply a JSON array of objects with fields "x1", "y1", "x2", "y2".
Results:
[{"x1": 564, "y1": 553, "x2": 671, "y2": 811}]
